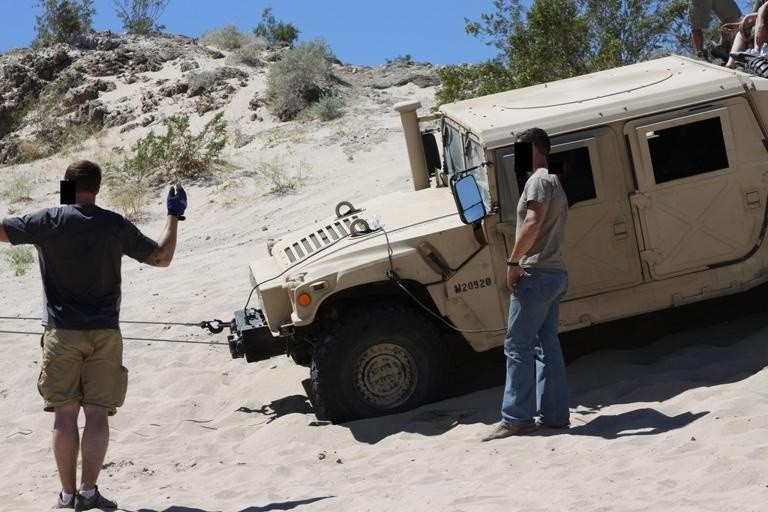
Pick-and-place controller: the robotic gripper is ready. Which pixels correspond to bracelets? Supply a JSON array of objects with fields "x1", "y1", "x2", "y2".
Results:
[{"x1": 506, "y1": 257, "x2": 520, "y2": 267}]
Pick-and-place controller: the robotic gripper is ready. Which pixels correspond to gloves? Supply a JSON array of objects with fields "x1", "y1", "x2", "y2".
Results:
[{"x1": 167, "y1": 184, "x2": 187, "y2": 220}]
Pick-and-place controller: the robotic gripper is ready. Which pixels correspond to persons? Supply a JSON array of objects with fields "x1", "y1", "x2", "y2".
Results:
[
  {"x1": 1, "y1": 160, "x2": 188, "y2": 511},
  {"x1": 719, "y1": 0, "x2": 768, "y2": 77},
  {"x1": 478, "y1": 128, "x2": 572, "y2": 443},
  {"x1": 687, "y1": 1, "x2": 743, "y2": 59}
]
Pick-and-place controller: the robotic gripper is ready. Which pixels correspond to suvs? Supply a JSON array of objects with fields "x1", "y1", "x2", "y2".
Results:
[{"x1": 222, "y1": 50, "x2": 768, "y2": 427}]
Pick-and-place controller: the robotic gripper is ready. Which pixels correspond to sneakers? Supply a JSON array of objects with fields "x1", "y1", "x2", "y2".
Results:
[
  {"x1": 482, "y1": 416, "x2": 568, "y2": 441},
  {"x1": 57, "y1": 485, "x2": 117, "y2": 511}
]
